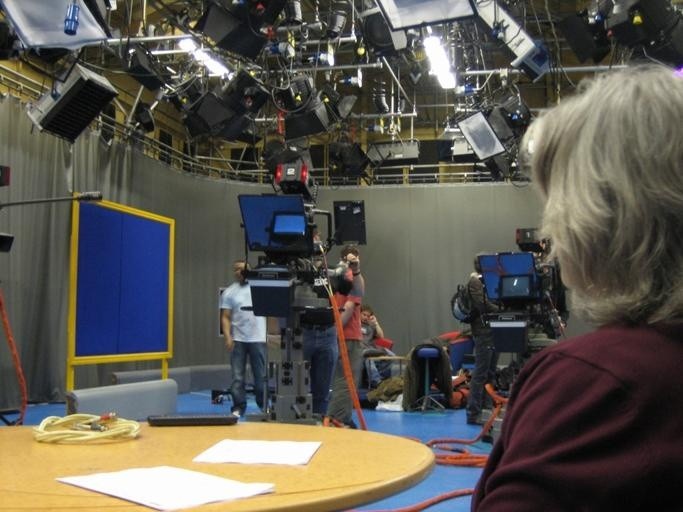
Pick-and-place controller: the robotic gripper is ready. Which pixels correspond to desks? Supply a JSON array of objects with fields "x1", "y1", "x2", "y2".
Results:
[
  {"x1": 367, "y1": 356, "x2": 407, "y2": 391},
  {"x1": 0, "y1": 421, "x2": 438, "y2": 511}
]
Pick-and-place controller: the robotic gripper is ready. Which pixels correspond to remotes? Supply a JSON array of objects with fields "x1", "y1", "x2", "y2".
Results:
[{"x1": 148, "y1": 413, "x2": 238, "y2": 426}]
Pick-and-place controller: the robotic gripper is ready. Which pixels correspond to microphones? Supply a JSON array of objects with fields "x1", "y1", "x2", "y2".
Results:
[{"x1": 0, "y1": 191, "x2": 102, "y2": 211}]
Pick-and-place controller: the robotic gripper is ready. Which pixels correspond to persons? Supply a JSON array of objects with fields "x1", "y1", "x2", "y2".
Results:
[
  {"x1": 220, "y1": 260, "x2": 269, "y2": 417},
  {"x1": 470, "y1": 62, "x2": 683, "y2": 512},
  {"x1": 301, "y1": 245, "x2": 390, "y2": 429},
  {"x1": 467, "y1": 252, "x2": 504, "y2": 425}
]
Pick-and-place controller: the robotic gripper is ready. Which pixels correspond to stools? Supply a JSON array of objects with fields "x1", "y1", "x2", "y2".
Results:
[{"x1": 412, "y1": 347, "x2": 445, "y2": 411}]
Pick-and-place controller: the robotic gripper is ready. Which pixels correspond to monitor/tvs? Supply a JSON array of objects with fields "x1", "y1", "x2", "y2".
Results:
[
  {"x1": 270, "y1": 211, "x2": 308, "y2": 236},
  {"x1": 499, "y1": 274, "x2": 534, "y2": 300}
]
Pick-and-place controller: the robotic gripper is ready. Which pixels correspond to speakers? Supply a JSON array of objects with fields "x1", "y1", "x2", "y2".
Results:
[{"x1": 29, "y1": 63, "x2": 120, "y2": 144}]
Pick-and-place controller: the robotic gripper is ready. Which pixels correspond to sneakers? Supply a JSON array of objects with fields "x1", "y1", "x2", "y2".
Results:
[
  {"x1": 232, "y1": 409, "x2": 243, "y2": 416},
  {"x1": 324, "y1": 416, "x2": 352, "y2": 427}
]
